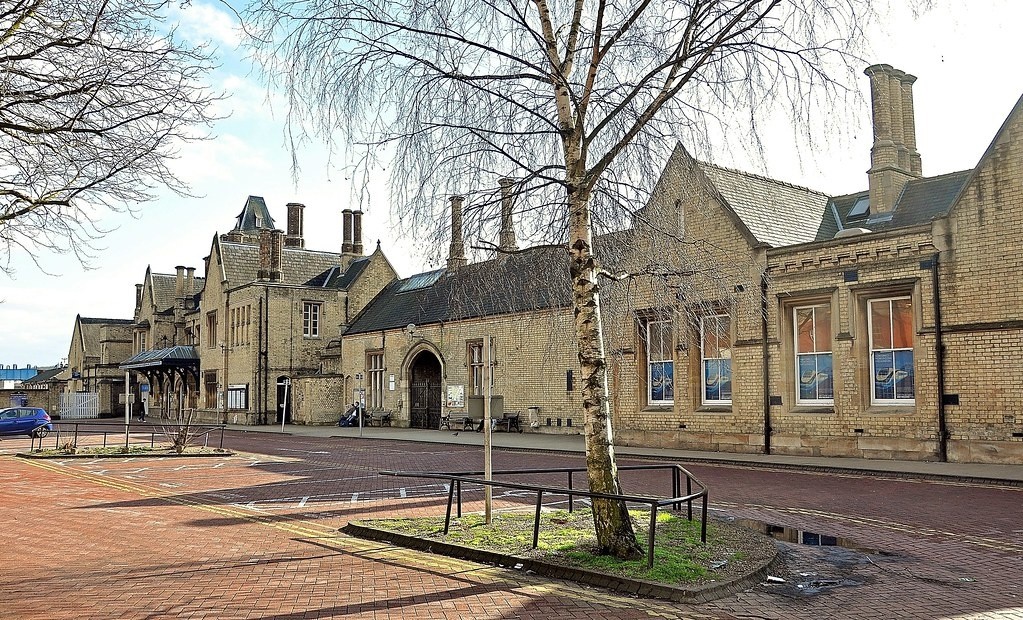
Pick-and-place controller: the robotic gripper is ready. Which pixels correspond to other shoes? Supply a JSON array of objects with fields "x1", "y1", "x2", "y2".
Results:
[
  {"x1": 143, "y1": 421, "x2": 146, "y2": 422},
  {"x1": 138, "y1": 420, "x2": 141, "y2": 422}
]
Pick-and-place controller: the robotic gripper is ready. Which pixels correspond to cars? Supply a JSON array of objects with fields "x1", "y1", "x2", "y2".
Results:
[{"x1": 0, "y1": 405, "x2": 54, "y2": 438}]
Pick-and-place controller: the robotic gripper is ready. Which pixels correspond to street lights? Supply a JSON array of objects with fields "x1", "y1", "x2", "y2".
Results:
[{"x1": 407, "y1": 323, "x2": 426, "y2": 342}]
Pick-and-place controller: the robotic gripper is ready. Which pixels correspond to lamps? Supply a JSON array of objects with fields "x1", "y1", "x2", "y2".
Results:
[
  {"x1": 218, "y1": 340, "x2": 234, "y2": 355},
  {"x1": 407, "y1": 324, "x2": 425, "y2": 342}
]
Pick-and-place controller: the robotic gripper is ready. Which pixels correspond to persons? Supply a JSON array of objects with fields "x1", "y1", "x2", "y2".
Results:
[{"x1": 137, "y1": 398, "x2": 146, "y2": 422}]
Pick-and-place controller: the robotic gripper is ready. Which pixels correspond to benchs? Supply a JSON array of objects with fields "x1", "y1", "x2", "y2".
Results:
[
  {"x1": 363, "y1": 408, "x2": 393, "y2": 429},
  {"x1": 440, "y1": 410, "x2": 473, "y2": 432},
  {"x1": 340, "y1": 415, "x2": 359, "y2": 426},
  {"x1": 493, "y1": 412, "x2": 520, "y2": 432}
]
